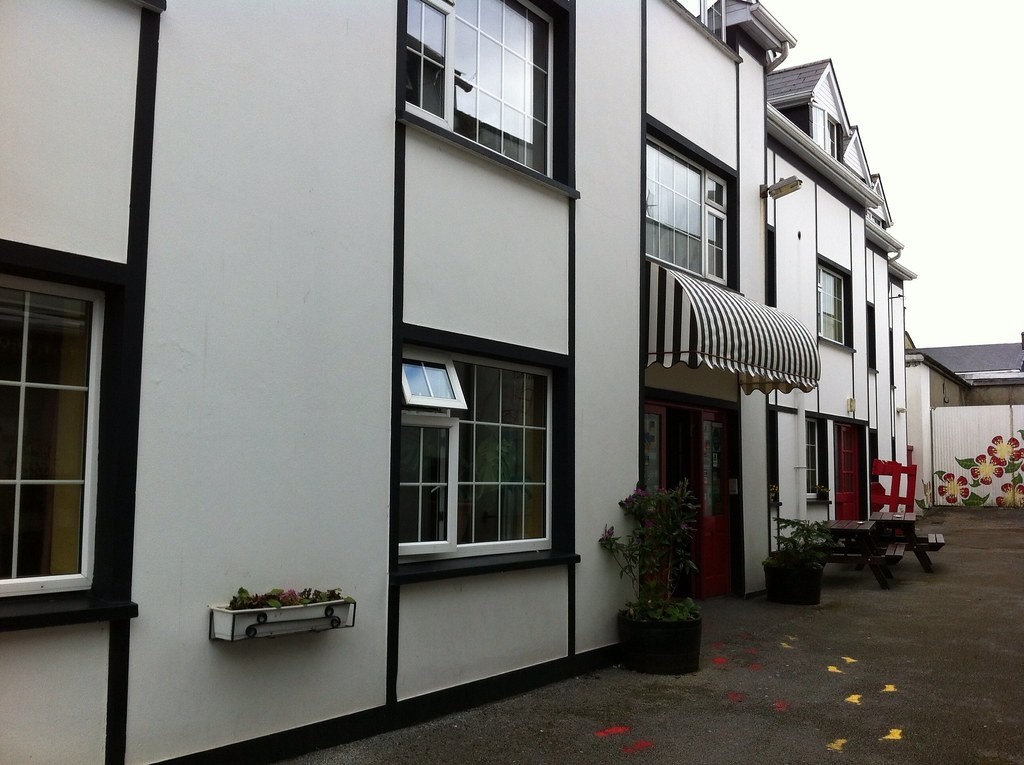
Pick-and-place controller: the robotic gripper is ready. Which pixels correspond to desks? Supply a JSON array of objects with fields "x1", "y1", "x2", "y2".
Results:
[
  {"x1": 817, "y1": 520, "x2": 894, "y2": 589},
  {"x1": 869, "y1": 511, "x2": 933, "y2": 573}
]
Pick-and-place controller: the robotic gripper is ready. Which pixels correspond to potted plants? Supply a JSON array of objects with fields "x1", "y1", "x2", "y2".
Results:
[
  {"x1": 761, "y1": 517, "x2": 845, "y2": 605},
  {"x1": 814, "y1": 485, "x2": 830, "y2": 500},
  {"x1": 210, "y1": 587, "x2": 350, "y2": 642}
]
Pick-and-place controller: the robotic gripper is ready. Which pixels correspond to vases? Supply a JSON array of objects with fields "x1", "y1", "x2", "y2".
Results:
[{"x1": 618, "y1": 613, "x2": 702, "y2": 675}]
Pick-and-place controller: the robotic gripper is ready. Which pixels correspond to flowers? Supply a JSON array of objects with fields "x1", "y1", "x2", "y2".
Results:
[{"x1": 600, "y1": 476, "x2": 704, "y2": 620}]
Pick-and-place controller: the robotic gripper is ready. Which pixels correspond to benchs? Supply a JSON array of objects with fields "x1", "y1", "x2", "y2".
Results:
[
  {"x1": 870, "y1": 543, "x2": 906, "y2": 589},
  {"x1": 914, "y1": 533, "x2": 946, "y2": 573}
]
